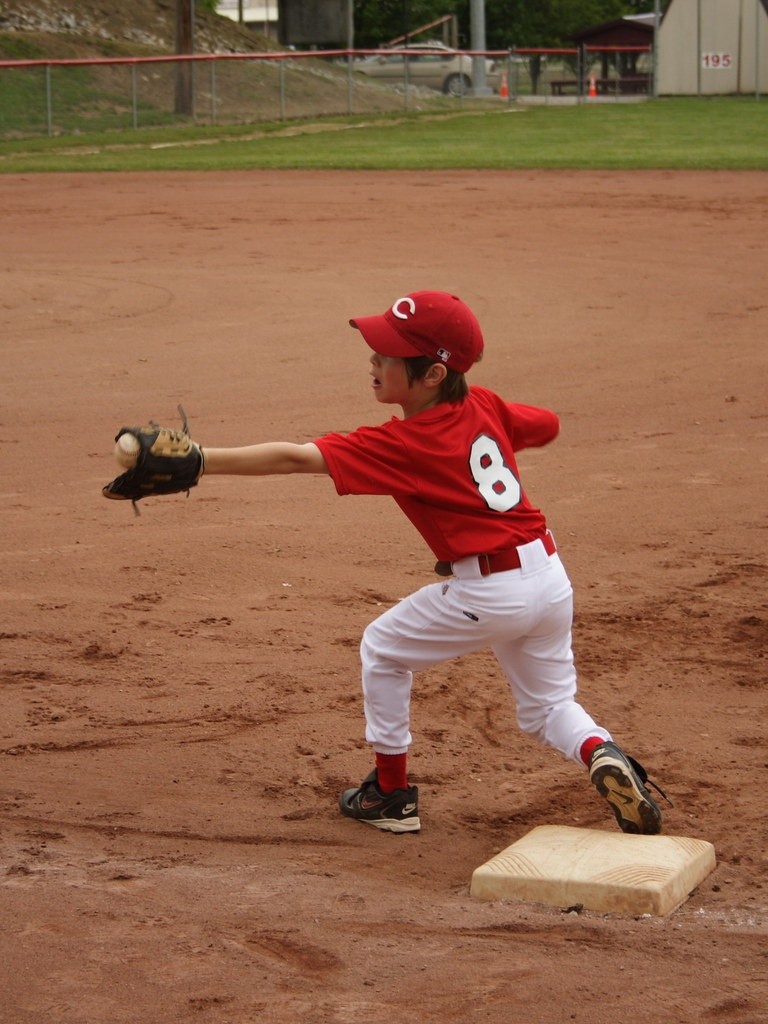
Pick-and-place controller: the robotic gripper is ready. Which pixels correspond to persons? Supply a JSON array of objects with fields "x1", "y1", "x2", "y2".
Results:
[{"x1": 116, "y1": 289, "x2": 663, "y2": 842}]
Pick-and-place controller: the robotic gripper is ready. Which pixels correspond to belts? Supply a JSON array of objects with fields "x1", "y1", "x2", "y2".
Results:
[{"x1": 434, "y1": 532, "x2": 557, "y2": 576}]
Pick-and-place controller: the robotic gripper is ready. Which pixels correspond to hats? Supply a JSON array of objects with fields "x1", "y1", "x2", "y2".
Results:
[{"x1": 348, "y1": 290, "x2": 485, "y2": 374}]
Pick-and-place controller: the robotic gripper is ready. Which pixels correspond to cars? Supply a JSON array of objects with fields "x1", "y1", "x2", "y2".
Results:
[{"x1": 347, "y1": 43, "x2": 496, "y2": 98}]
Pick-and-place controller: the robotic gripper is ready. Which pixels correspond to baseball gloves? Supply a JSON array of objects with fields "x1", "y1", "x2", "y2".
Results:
[{"x1": 101, "y1": 403, "x2": 205, "y2": 515}]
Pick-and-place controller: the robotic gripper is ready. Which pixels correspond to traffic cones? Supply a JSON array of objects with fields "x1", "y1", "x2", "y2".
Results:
[
  {"x1": 588, "y1": 71, "x2": 598, "y2": 97},
  {"x1": 499, "y1": 70, "x2": 511, "y2": 103}
]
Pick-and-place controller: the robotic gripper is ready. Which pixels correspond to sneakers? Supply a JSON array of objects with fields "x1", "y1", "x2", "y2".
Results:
[
  {"x1": 338, "y1": 767, "x2": 421, "y2": 833},
  {"x1": 588, "y1": 741, "x2": 674, "y2": 835}
]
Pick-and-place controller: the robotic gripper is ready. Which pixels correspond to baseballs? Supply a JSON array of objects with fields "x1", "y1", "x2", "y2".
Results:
[{"x1": 112, "y1": 433, "x2": 139, "y2": 465}]
